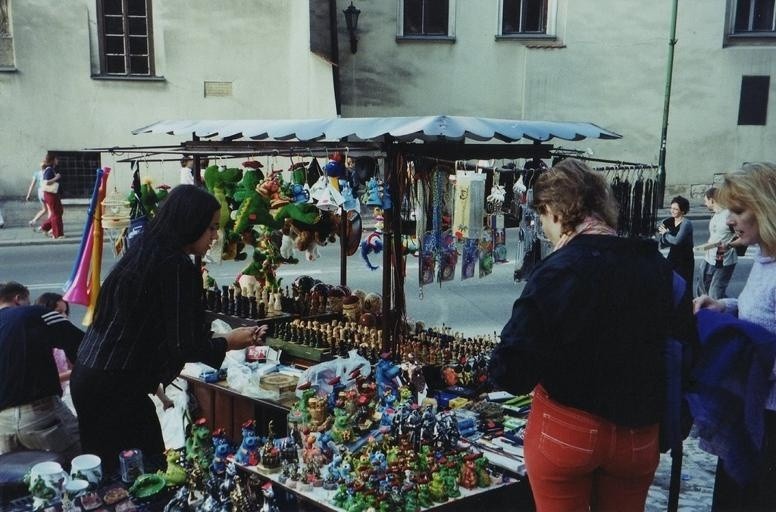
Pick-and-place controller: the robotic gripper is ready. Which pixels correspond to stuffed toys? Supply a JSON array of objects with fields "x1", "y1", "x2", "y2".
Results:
[{"x1": 128, "y1": 162, "x2": 319, "y2": 288}]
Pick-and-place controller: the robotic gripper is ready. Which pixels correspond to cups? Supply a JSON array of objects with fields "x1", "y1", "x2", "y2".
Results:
[
  {"x1": 119, "y1": 449, "x2": 145, "y2": 483},
  {"x1": 23, "y1": 462, "x2": 68, "y2": 504},
  {"x1": 71, "y1": 454, "x2": 102, "y2": 493},
  {"x1": 65, "y1": 480, "x2": 88, "y2": 501}
]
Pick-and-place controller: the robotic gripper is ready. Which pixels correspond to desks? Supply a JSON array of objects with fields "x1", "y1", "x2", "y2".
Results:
[{"x1": 177, "y1": 348, "x2": 535, "y2": 512}]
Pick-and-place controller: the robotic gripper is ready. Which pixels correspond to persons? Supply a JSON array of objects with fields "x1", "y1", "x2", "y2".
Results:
[
  {"x1": 691, "y1": 162, "x2": 776, "y2": 511},
  {"x1": 201, "y1": 156, "x2": 210, "y2": 179},
  {"x1": 181, "y1": 158, "x2": 194, "y2": 185},
  {"x1": 699, "y1": 188, "x2": 750, "y2": 299},
  {"x1": 489, "y1": 157, "x2": 689, "y2": 512},
  {"x1": 71, "y1": 184, "x2": 269, "y2": 474},
  {"x1": 32, "y1": 293, "x2": 73, "y2": 391},
  {"x1": 658, "y1": 196, "x2": 694, "y2": 291},
  {"x1": 38, "y1": 155, "x2": 63, "y2": 240},
  {"x1": 25, "y1": 160, "x2": 51, "y2": 233},
  {"x1": 1, "y1": 282, "x2": 85, "y2": 463}
]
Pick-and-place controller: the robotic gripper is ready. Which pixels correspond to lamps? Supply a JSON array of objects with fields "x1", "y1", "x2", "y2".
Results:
[{"x1": 342, "y1": 1, "x2": 360, "y2": 54}]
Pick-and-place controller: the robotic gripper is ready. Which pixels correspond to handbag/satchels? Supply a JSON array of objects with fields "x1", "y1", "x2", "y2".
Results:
[
  {"x1": 663, "y1": 217, "x2": 675, "y2": 232},
  {"x1": 660, "y1": 338, "x2": 694, "y2": 455},
  {"x1": 40, "y1": 179, "x2": 58, "y2": 194},
  {"x1": 731, "y1": 235, "x2": 747, "y2": 257}
]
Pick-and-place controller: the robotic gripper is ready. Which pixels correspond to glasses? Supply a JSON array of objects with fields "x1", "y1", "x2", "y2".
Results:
[{"x1": 670, "y1": 207, "x2": 680, "y2": 211}]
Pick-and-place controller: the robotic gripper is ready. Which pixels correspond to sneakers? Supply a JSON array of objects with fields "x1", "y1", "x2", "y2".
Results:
[
  {"x1": 47, "y1": 229, "x2": 52, "y2": 238},
  {"x1": 37, "y1": 226, "x2": 48, "y2": 238},
  {"x1": 53, "y1": 236, "x2": 65, "y2": 240},
  {"x1": 27, "y1": 220, "x2": 35, "y2": 233}
]
